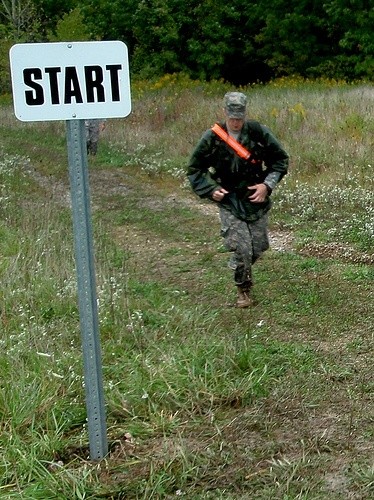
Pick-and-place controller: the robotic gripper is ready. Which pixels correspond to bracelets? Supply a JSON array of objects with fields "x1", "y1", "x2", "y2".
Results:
[{"x1": 263, "y1": 183, "x2": 273, "y2": 197}]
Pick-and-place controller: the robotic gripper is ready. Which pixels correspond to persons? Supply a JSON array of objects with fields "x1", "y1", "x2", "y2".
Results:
[
  {"x1": 85, "y1": 118, "x2": 106, "y2": 156},
  {"x1": 186, "y1": 92, "x2": 290, "y2": 308}
]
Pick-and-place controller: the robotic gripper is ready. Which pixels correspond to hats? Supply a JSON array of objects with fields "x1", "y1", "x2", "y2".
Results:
[{"x1": 222, "y1": 91, "x2": 247, "y2": 118}]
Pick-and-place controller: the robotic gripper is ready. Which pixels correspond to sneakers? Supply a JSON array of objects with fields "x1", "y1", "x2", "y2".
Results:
[{"x1": 235, "y1": 287, "x2": 255, "y2": 307}]
